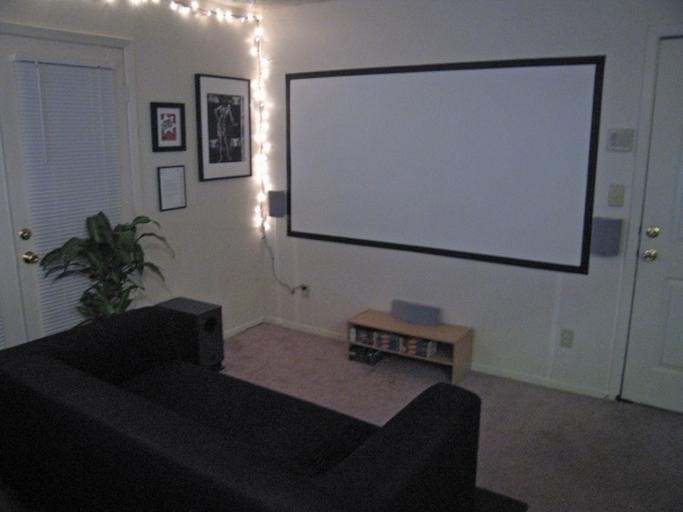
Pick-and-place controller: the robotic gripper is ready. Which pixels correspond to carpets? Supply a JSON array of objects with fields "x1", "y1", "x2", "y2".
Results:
[{"x1": 222, "y1": 321, "x2": 682, "y2": 511}]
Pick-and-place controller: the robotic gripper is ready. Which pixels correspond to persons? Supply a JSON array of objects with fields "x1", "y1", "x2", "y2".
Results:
[{"x1": 213, "y1": 98, "x2": 239, "y2": 164}]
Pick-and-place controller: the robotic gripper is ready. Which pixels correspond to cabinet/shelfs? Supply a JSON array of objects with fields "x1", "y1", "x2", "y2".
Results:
[{"x1": 346, "y1": 311, "x2": 474, "y2": 383}]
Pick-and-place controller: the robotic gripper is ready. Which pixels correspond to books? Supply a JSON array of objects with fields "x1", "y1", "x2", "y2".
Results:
[
  {"x1": 355, "y1": 326, "x2": 400, "y2": 353},
  {"x1": 402, "y1": 335, "x2": 438, "y2": 358}
]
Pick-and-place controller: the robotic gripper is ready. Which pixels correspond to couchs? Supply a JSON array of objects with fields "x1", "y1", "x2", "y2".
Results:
[{"x1": 0, "y1": 307, "x2": 481, "y2": 512}]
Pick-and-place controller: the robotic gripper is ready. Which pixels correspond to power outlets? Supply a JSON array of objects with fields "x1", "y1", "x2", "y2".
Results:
[
  {"x1": 300, "y1": 284, "x2": 313, "y2": 298},
  {"x1": 608, "y1": 184, "x2": 625, "y2": 207},
  {"x1": 560, "y1": 328, "x2": 576, "y2": 349}
]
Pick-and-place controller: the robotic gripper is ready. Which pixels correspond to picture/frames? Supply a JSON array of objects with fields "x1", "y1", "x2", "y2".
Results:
[
  {"x1": 150, "y1": 102, "x2": 187, "y2": 152},
  {"x1": 157, "y1": 165, "x2": 187, "y2": 212},
  {"x1": 195, "y1": 73, "x2": 252, "y2": 182}
]
